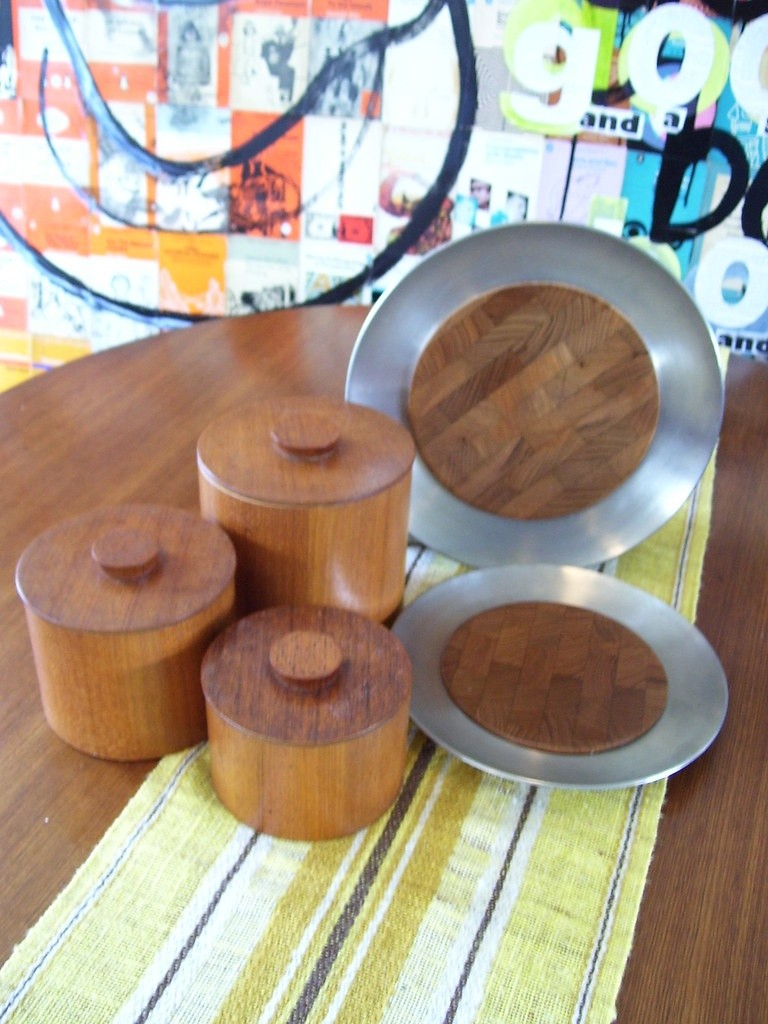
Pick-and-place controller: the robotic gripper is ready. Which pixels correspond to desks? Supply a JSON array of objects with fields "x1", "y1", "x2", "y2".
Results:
[{"x1": 0, "y1": 305, "x2": 768, "y2": 1024}]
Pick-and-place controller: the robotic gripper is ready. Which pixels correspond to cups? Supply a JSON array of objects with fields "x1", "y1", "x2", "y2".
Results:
[
  {"x1": 199, "y1": 603, "x2": 414, "y2": 841},
  {"x1": 14, "y1": 502, "x2": 236, "y2": 763},
  {"x1": 195, "y1": 396, "x2": 416, "y2": 624}
]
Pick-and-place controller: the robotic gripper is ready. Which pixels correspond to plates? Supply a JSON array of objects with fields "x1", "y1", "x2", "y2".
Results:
[
  {"x1": 389, "y1": 563, "x2": 729, "y2": 791},
  {"x1": 343, "y1": 223, "x2": 725, "y2": 567}
]
